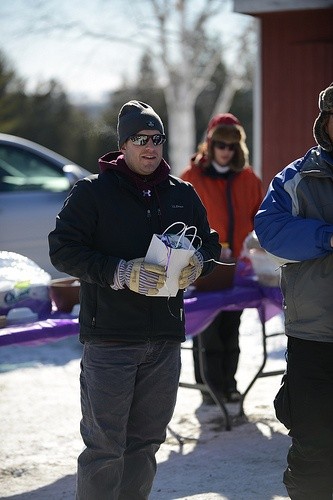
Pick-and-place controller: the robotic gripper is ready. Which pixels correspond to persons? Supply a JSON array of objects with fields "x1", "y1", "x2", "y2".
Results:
[
  {"x1": 253, "y1": 83, "x2": 333, "y2": 500},
  {"x1": 177, "y1": 111, "x2": 268, "y2": 404},
  {"x1": 48, "y1": 99, "x2": 221, "y2": 500}
]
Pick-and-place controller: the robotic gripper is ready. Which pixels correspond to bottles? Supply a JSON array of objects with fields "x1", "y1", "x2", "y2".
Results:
[{"x1": 221, "y1": 242, "x2": 232, "y2": 259}]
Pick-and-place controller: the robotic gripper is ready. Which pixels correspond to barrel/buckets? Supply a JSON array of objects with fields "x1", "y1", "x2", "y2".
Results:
[{"x1": 49, "y1": 277, "x2": 80, "y2": 313}]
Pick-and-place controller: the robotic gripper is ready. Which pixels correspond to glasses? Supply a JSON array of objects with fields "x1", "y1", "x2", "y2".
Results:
[
  {"x1": 214, "y1": 140, "x2": 238, "y2": 151},
  {"x1": 127, "y1": 133, "x2": 167, "y2": 146}
]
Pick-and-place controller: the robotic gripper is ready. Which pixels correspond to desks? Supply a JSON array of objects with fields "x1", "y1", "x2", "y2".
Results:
[{"x1": 0, "y1": 285, "x2": 286, "y2": 447}]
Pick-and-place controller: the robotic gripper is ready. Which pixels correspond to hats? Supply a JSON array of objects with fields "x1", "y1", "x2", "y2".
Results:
[
  {"x1": 313, "y1": 83, "x2": 333, "y2": 152},
  {"x1": 195, "y1": 113, "x2": 249, "y2": 172},
  {"x1": 116, "y1": 100, "x2": 166, "y2": 149}
]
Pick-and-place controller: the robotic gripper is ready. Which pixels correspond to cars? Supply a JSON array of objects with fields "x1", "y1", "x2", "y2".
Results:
[{"x1": 0, "y1": 131, "x2": 98, "y2": 282}]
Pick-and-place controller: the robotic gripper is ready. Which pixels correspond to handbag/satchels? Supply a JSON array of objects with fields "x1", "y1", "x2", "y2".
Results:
[{"x1": 144, "y1": 222, "x2": 197, "y2": 296}]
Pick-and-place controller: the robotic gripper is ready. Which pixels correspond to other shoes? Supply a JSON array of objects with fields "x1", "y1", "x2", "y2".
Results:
[
  {"x1": 225, "y1": 390, "x2": 241, "y2": 404},
  {"x1": 203, "y1": 393, "x2": 225, "y2": 405}
]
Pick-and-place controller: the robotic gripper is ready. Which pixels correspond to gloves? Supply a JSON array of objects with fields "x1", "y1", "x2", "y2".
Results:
[
  {"x1": 179, "y1": 251, "x2": 204, "y2": 289},
  {"x1": 110, "y1": 259, "x2": 166, "y2": 297}
]
